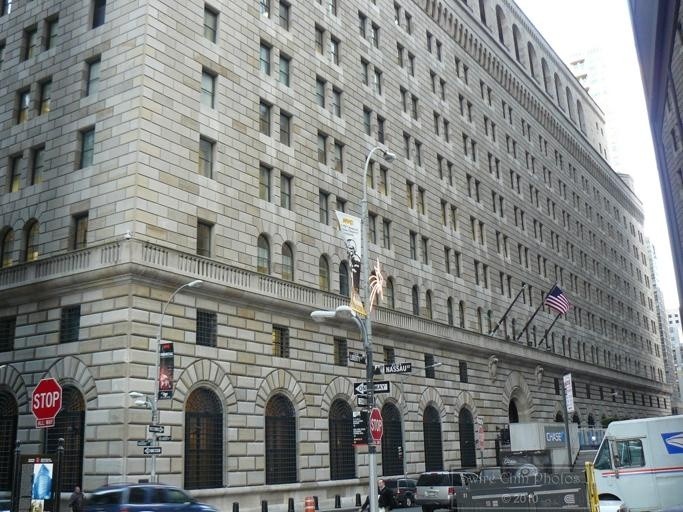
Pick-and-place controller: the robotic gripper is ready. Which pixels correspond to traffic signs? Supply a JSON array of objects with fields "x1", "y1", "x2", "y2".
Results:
[
  {"x1": 142, "y1": 447, "x2": 161, "y2": 454},
  {"x1": 347, "y1": 351, "x2": 367, "y2": 365},
  {"x1": 155, "y1": 435, "x2": 172, "y2": 441},
  {"x1": 352, "y1": 381, "x2": 391, "y2": 395},
  {"x1": 136, "y1": 439, "x2": 152, "y2": 445},
  {"x1": 147, "y1": 425, "x2": 164, "y2": 433},
  {"x1": 372, "y1": 362, "x2": 411, "y2": 374}
]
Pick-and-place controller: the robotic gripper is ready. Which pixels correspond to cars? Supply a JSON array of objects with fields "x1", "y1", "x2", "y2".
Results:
[
  {"x1": 80, "y1": 483, "x2": 220, "y2": 511},
  {"x1": 379, "y1": 478, "x2": 417, "y2": 507}
]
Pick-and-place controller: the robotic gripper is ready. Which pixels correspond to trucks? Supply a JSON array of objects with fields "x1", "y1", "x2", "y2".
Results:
[{"x1": 587, "y1": 414, "x2": 683, "y2": 512}]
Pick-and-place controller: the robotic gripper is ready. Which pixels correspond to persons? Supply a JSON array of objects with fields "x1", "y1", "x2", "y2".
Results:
[
  {"x1": 69, "y1": 487, "x2": 84, "y2": 512},
  {"x1": 359, "y1": 480, "x2": 393, "y2": 512}
]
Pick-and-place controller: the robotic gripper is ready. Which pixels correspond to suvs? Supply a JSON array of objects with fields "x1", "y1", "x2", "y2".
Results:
[{"x1": 414, "y1": 471, "x2": 481, "y2": 510}]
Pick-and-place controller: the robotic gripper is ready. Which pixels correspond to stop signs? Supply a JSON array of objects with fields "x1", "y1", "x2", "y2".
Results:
[
  {"x1": 30, "y1": 377, "x2": 61, "y2": 420},
  {"x1": 369, "y1": 407, "x2": 384, "y2": 441}
]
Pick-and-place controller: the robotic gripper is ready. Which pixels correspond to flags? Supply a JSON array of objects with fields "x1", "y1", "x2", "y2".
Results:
[{"x1": 544, "y1": 285, "x2": 569, "y2": 315}]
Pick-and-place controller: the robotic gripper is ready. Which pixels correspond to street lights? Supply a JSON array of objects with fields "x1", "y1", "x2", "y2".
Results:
[
  {"x1": 129, "y1": 277, "x2": 203, "y2": 485},
  {"x1": 309, "y1": 144, "x2": 399, "y2": 512},
  {"x1": 397, "y1": 362, "x2": 441, "y2": 476},
  {"x1": 587, "y1": 391, "x2": 620, "y2": 449}
]
